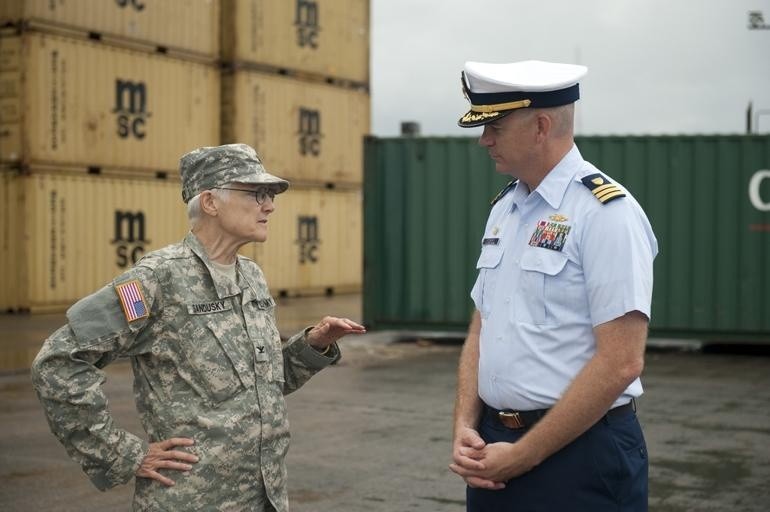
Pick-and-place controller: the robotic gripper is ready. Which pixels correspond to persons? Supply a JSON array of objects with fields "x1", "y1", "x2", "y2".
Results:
[
  {"x1": 446, "y1": 59, "x2": 658, "y2": 512},
  {"x1": 29, "y1": 142, "x2": 367, "y2": 512}
]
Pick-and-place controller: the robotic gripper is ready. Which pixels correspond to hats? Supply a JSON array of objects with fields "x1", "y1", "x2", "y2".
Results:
[
  {"x1": 178, "y1": 144, "x2": 289, "y2": 204},
  {"x1": 458, "y1": 60, "x2": 588, "y2": 128}
]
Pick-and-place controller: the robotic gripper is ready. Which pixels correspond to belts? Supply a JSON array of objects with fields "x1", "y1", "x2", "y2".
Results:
[{"x1": 484, "y1": 400, "x2": 633, "y2": 428}]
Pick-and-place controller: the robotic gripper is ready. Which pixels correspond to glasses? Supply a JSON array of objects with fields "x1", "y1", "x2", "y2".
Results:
[{"x1": 209, "y1": 186, "x2": 275, "y2": 205}]
